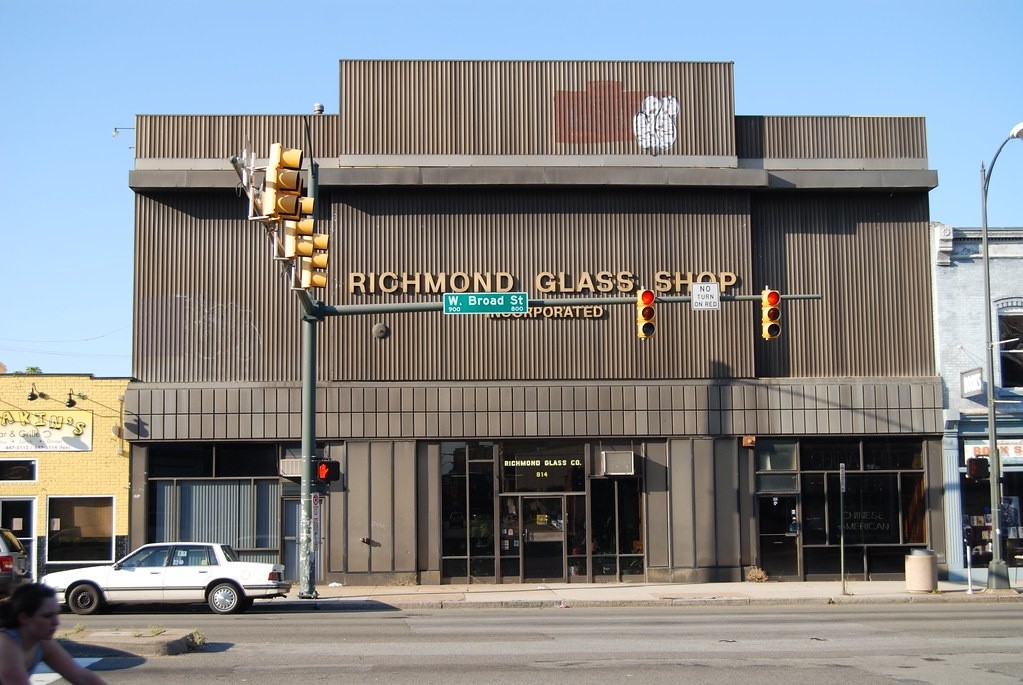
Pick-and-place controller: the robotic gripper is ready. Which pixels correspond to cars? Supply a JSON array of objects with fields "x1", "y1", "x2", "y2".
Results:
[
  {"x1": 40, "y1": 540, "x2": 292, "y2": 615},
  {"x1": 0, "y1": 527, "x2": 29, "y2": 599}
]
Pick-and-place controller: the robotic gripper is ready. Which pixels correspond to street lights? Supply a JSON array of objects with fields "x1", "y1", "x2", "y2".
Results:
[{"x1": 978, "y1": 121, "x2": 1023, "y2": 589}]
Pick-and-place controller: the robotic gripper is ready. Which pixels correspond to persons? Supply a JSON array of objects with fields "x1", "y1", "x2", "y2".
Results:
[
  {"x1": 1000, "y1": 498, "x2": 1018, "y2": 527},
  {"x1": 0, "y1": 584, "x2": 109, "y2": 685}
]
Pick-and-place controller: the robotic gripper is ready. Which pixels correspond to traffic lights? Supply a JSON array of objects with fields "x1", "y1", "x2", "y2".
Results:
[
  {"x1": 300, "y1": 233, "x2": 330, "y2": 290},
  {"x1": 266, "y1": 142, "x2": 305, "y2": 221},
  {"x1": 316, "y1": 460, "x2": 341, "y2": 481},
  {"x1": 283, "y1": 195, "x2": 317, "y2": 258},
  {"x1": 637, "y1": 289, "x2": 657, "y2": 340},
  {"x1": 760, "y1": 289, "x2": 782, "y2": 340}
]
